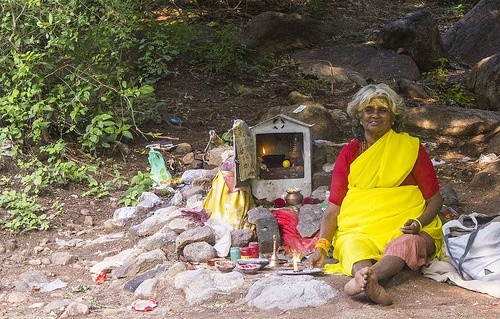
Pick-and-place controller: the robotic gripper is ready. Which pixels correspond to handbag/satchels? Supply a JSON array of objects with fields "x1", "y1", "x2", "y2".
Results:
[{"x1": 442, "y1": 212, "x2": 500, "y2": 281}]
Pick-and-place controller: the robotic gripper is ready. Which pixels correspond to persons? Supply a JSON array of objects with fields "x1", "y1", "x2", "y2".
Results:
[{"x1": 303, "y1": 83, "x2": 445, "y2": 306}]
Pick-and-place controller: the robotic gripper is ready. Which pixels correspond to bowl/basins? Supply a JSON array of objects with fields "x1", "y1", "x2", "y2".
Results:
[
  {"x1": 238, "y1": 263, "x2": 261, "y2": 273},
  {"x1": 215, "y1": 261, "x2": 236, "y2": 272},
  {"x1": 237, "y1": 258, "x2": 269, "y2": 268}
]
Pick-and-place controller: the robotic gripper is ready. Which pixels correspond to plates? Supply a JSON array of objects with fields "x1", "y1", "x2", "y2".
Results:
[{"x1": 276, "y1": 267, "x2": 322, "y2": 275}]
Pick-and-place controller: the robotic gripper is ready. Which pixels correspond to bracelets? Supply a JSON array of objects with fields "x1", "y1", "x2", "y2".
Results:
[{"x1": 414, "y1": 218, "x2": 423, "y2": 233}]
